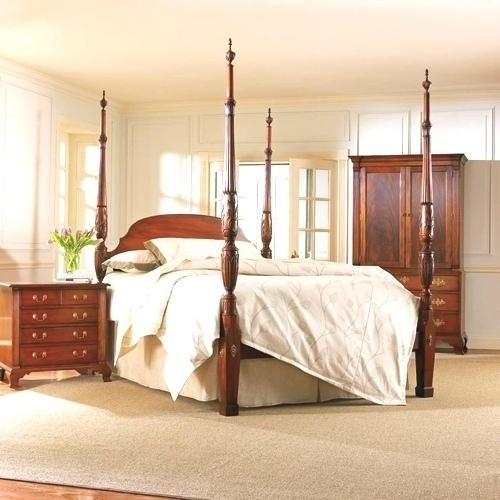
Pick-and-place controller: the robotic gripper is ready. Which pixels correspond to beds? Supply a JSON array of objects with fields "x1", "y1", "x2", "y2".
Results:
[{"x1": 94, "y1": 39, "x2": 435, "y2": 416}]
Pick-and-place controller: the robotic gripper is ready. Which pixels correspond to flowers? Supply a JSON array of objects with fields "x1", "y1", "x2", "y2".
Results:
[{"x1": 48, "y1": 227, "x2": 103, "y2": 274}]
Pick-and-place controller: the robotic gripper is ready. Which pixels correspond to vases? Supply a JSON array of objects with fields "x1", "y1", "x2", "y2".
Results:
[{"x1": 62, "y1": 252, "x2": 80, "y2": 280}]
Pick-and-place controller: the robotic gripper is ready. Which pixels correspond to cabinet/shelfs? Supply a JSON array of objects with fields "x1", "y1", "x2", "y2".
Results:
[
  {"x1": 348, "y1": 154, "x2": 469, "y2": 356},
  {"x1": 0, "y1": 283, "x2": 111, "y2": 388}
]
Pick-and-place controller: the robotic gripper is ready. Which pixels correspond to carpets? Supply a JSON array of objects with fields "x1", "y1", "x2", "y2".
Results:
[{"x1": 0, "y1": 351, "x2": 500, "y2": 500}]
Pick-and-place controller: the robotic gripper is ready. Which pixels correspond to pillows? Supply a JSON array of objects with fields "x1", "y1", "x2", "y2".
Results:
[{"x1": 102, "y1": 237, "x2": 262, "y2": 274}]
